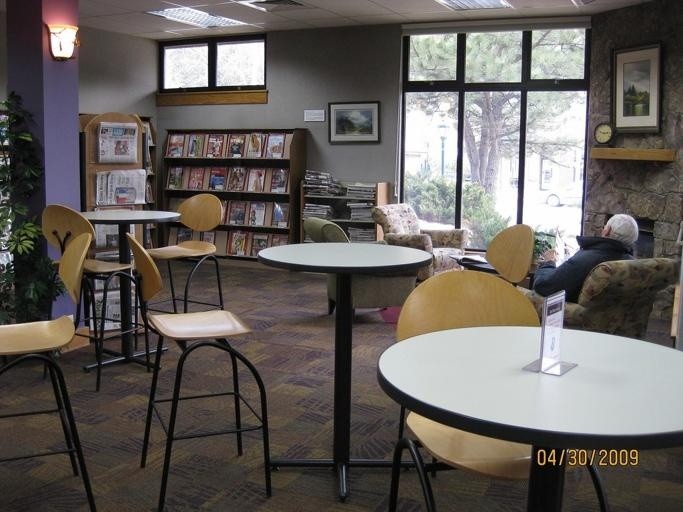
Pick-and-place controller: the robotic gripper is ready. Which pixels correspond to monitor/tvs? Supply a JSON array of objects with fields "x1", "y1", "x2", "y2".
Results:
[
  {"x1": 451, "y1": 254, "x2": 487, "y2": 264},
  {"x1": 164, "y1": 133, "x2": 290, "y2": 258},
  {"x1": 302, "y1": 169, "x2": 375, "y2": 243}
]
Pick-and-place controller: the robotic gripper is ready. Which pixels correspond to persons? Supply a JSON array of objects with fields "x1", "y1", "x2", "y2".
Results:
[{"x1": 534, "y1": 213, "x2": 641, "y2": 303}]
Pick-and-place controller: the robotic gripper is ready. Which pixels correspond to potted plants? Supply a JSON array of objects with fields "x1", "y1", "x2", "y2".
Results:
[
  {"x1": 611, "y1": 39, "x2": 666, "y2": 137},
  {"x1": 324, "y1": 99, "x2": 382, "y2": 147}
]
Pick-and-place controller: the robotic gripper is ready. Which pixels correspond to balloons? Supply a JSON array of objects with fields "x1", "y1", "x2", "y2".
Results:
[{"x1": 592, "y1": 120, "x2": 617, "y2": 150}]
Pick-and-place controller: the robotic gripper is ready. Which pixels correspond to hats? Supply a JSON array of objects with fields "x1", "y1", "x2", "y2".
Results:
[
  {"x1": 435, "y1": 119, "x2": 451, "y2": 180},
  {"x1": 541, "y1": 178, "x2": 584, "y2": 210}
]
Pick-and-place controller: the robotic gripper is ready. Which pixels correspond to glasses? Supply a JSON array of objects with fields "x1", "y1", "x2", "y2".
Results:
[{"x1": 43, "y1": 22, "x2": 81, "y2": 64}]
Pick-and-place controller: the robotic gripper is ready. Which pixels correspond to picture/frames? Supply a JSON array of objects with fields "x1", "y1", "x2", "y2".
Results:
[
  {"x1": 74, "y1": 207, "x2": 187, "y2": 374},
  {"x1": 251, "y1": 240, "x2": 433, "y2": 502},
  {"x1": 372, "y1": 323, "x2": 681, "y2": 511}
]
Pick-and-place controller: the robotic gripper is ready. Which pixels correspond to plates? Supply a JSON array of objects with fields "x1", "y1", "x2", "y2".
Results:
[
  {"x1": 76, "y1": 112, "x2": 159, "y2": 249},
  {"x1": 155, "y1": 124, "x2": 310, "y2": 263},
  {"x1": 296, "y1": 177, "x2": 390, "y2": 246}
]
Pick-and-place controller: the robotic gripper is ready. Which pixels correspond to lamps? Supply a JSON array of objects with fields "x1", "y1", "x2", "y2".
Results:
[
  {"x1": 300, "y1": 213, "x2": 419, "y2": 320},
  {"x1": 380, "y1": 270, "x2": 613, "y2": 511},
  {"x1": 133, "y1": 193, "x2": 226, "y2": 315},
  {"x1": 512, "y1": 255, "x2": 679, "y2": 341},
  {"x1": 0, "y1": 226, "x2": 100, "y2": 510},
  {"x1": 36, "y1": 202, "x2": 151, "y2": 394},
  {"x1": 121, "y1": 230, "x2": 273, "y2": 511},
  {"x1": 482, "y1": 221, "x2": 536, "y2": 290},
  {"x1": 365, "y1": 200, "x2": 468, "y2": 285}
]
